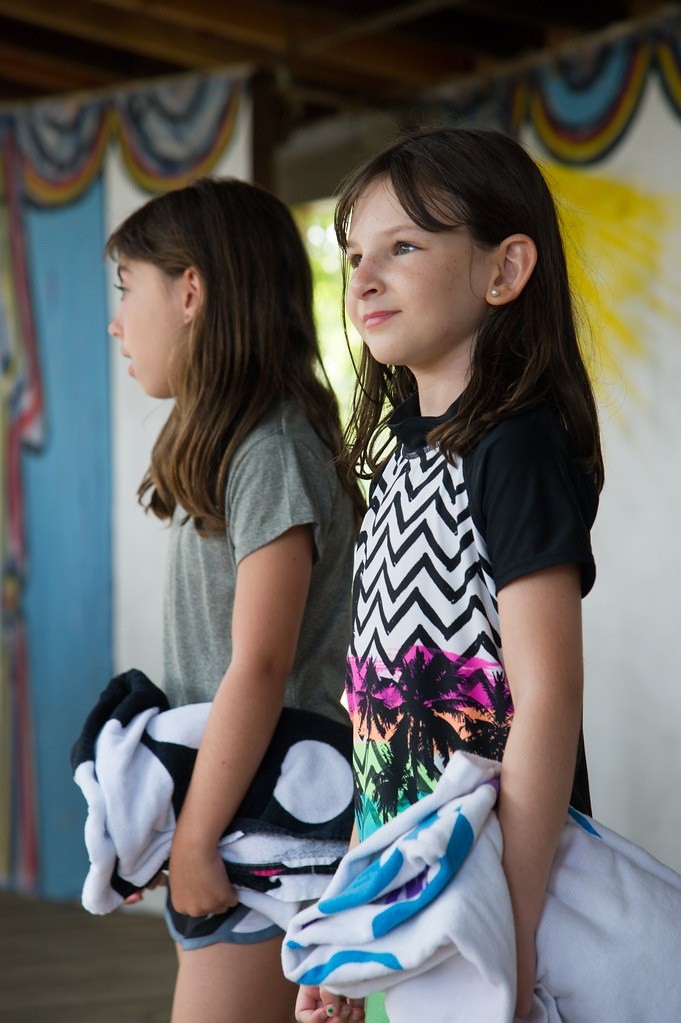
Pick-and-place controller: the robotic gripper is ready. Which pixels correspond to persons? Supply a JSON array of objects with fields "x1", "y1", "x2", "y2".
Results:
[
  {"x1": 285, "y1": 113, "x2": 604, "y2": 1021},
  {"x1": 84, "y1": 174, "x2": 364, "y2": 1021}
]
